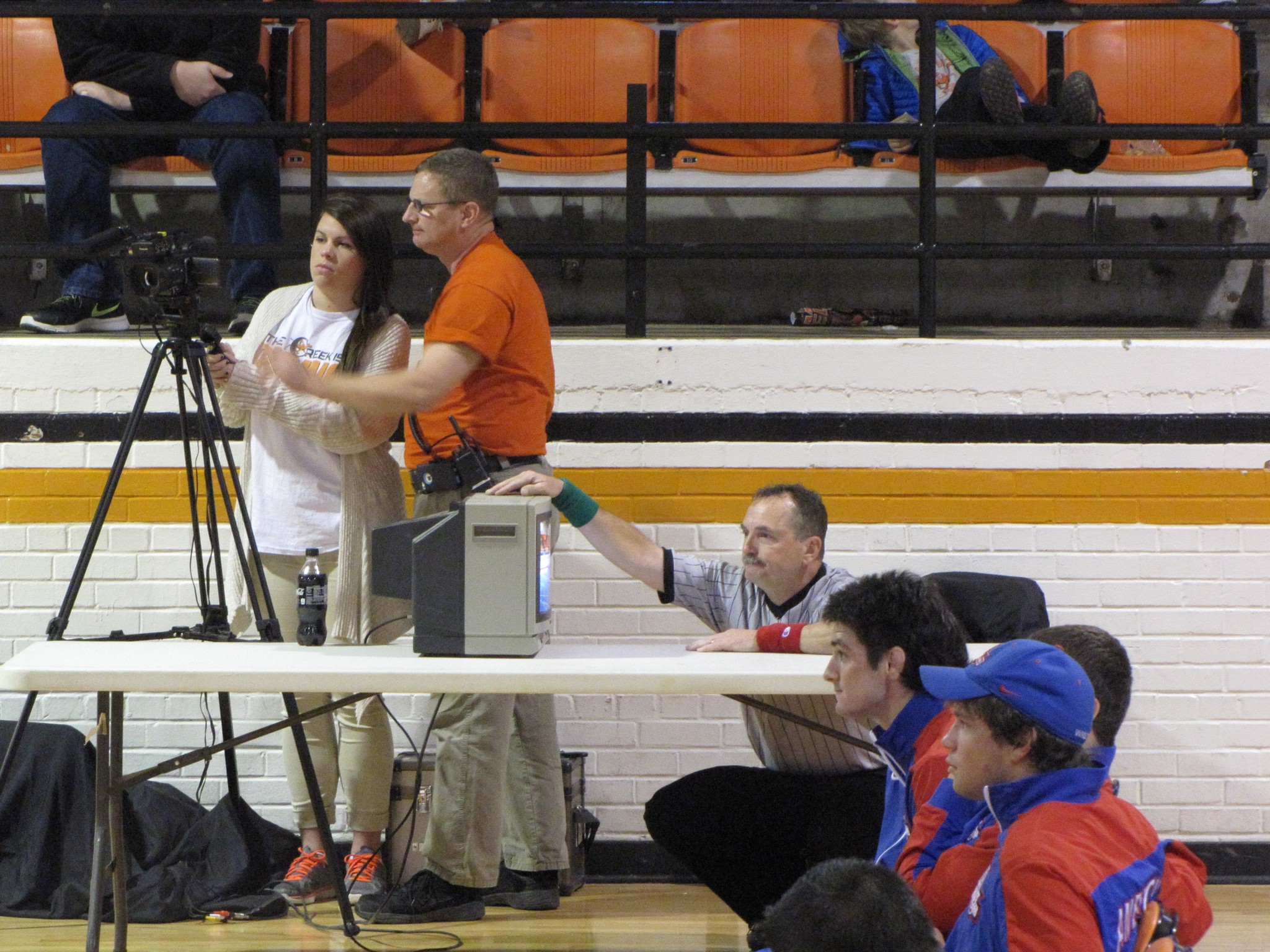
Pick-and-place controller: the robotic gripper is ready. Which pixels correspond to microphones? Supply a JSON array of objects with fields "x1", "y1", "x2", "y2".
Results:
[{"x1": 79, "y1": 223, "x2": 141, "y2": 255}]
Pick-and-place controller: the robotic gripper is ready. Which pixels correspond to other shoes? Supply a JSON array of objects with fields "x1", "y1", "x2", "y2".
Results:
[
  {"x1": 1059, "y1": 71, "x2": 1101, "y2": 159},
  {"x1": 979, "y1": 57, "x2": 1026, "y2": 125}
]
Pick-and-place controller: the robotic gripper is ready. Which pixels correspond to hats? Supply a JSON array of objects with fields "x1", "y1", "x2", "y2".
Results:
[{"x1": 918, "y1": 639, "x2": 1096, "y2": 745}]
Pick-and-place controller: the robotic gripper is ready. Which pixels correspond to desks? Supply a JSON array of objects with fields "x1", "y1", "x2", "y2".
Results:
[{"x1": 0, "y1": 642, "x2": 1002, "y2": 951}]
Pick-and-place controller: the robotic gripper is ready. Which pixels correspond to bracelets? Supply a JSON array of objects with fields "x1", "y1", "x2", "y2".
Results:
[
  {"x1": 552, "y1": 477, "x2": 600, "y2": 528},
  {"x1": 757, "y1": 623, "x2": 807, "y2": 653}
]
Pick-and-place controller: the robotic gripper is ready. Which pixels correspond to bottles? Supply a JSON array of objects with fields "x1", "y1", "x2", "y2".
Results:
[{"x1": 296, "y1": 549, "x2": 328, "y2": 646}]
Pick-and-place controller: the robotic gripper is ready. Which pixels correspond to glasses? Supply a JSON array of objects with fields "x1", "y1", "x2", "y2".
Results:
[{"x1": 406, "y1": 196, "x2": 468, "y2": 212}]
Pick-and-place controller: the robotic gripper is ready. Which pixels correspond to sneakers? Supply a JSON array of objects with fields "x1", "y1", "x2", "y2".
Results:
[
  {"x1": 342, "y1": 846, "x2": 386, "y2": 904},
  {"x1": 481, "y1": 861, "x2": 560, "y2": 909},
  {"x1": 226, "y1": 296, "x2": 262, "y2": 333},
  {"x1": 353, "y1": 869, "x2": 484, "y2": 923},
  {"x1": 20, "y1": 294, "x2": 131, "y2": 332},
  {"x1": 271, "y1": 845, "x2": 336, "y2": 903}
]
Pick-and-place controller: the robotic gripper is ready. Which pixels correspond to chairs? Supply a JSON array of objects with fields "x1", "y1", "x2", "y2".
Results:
[{"x1": 0, "y1": 16, "x2": 1254, "y2": 190}]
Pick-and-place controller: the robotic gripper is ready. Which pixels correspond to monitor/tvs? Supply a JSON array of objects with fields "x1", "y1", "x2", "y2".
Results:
[{"x1": 370, "y1": 494, "x2": 553, "y2": 658}]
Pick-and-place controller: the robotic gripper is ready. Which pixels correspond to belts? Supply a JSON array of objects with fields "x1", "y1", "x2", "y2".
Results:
[{"x1": 482, "y1": 453, "x2": 541, "y2": 471}]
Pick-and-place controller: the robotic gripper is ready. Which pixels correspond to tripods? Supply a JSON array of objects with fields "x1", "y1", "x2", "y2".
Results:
[{"x1": 1, "y1": 326, "x2": 361, "y2": 937}]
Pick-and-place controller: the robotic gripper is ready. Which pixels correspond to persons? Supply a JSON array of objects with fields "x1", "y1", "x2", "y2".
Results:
[
  {"x1": 483, "y1": 467, "x2": 1214, "y2": 952},
  {"x1": 207, "y1": 197, "x2": 412, "y2": 907},
  {"x1": 20, "y1": 2, "x2": 279, "y2": 333},
  {"x1": 837, "y1": 17, "x2": 1110, "y2": 174},
  {"x1": 255, "y1": 149, "x2": 572, "y2": 923}
]
